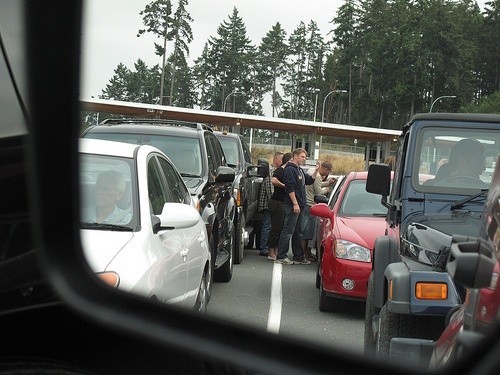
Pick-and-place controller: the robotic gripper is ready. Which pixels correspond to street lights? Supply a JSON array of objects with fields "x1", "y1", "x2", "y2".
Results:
[
  {"x1": 430, "y1": 95, "x2": 456, "y2": 113},
  {"x1": 221, "y1": 92, "x2": 247, "y2": 131},
  {"x1": 322, "y1": 89, "x2": 347, "y2": 123}
]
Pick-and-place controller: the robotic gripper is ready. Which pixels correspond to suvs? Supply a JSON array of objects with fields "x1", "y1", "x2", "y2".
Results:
[
  {"x1": 363, "y1": 113, "x2": 500, "y2": 361},
  {"x1": 82, "y1": 116, "x2": 238, "y2": 284}
]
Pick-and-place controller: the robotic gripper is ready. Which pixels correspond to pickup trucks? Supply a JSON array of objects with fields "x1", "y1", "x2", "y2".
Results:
[{"x1": 212, "y1": 131, "x2": 270, "y2": 265}]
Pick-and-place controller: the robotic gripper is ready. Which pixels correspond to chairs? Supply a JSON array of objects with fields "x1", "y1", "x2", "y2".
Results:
[{"x1": 173, "y1": 143, "x2": 200, "y2": 175}]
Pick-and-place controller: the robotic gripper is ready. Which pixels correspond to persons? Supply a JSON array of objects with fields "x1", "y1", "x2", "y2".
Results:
[
  {"x1": 273, "y1": 147, "x2": 322, "y2": 265},
  {"x1": 429, "y1": 139, "x2": 489, "y2": 198},
  {"x1": 80, "y1": 170, "x2": 132, "y2": 224},
  {"x1": 304, "y1": 168, "x2": 332, "y2": 259},
  {"x1": 267, "y1": 152, "x2": 322, "y2": 261},
  {"x1": 256, "y1": 150, "x2": 285, "y2": 256},
  {"x1": 297, "y1": 161, "x2": 336, "y2": 262},
  {"x1": 418, "y1": 157, "x2": 456, "y2": 189}
]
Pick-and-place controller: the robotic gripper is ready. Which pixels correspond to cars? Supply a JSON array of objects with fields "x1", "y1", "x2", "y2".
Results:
[
  {"x1": 307, "y1": 168, "x2": 433, "y2": 313},
  {"x1": 79, "y1": 135, "x2": 213, "y2": 315},
  {"x1": 428, "y1": 146, "x2": 500, "y2": 370},
  {"x1": 299, "y1": 159, "x2": 345, "y2": 256}
]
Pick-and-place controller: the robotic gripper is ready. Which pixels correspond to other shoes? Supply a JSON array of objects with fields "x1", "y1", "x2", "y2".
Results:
[{"x1": 247, "y1": 241, "x2": 316, "y2": 265}]
